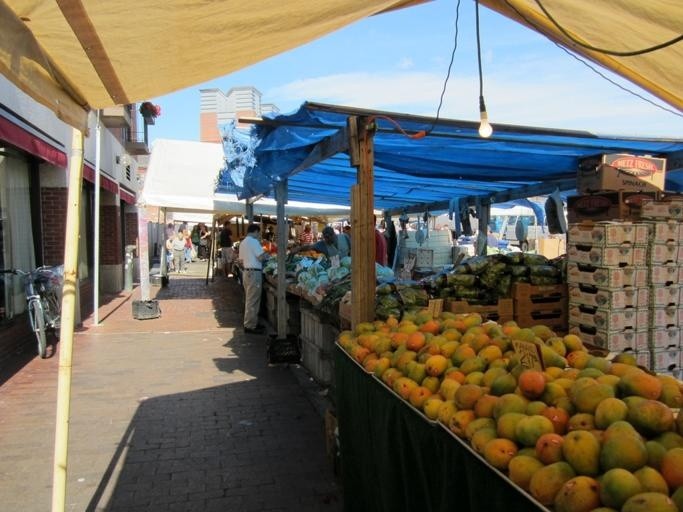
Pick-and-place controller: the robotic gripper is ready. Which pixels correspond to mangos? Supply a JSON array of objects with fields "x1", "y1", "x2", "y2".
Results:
[{"x1": 337, "y1": 309, "x2": 683, "y2": 511}]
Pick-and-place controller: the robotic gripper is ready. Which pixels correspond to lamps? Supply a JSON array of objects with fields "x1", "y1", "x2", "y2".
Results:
[{"x1": 478, "y1": 96, "x2": 493, "y2": 138}]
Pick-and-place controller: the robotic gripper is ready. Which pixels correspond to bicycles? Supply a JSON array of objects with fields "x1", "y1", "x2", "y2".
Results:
[{"x1": 0, "y1": 263, "x2": 61, "y2": 359}]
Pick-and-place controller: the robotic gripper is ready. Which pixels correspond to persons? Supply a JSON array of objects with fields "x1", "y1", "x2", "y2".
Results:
[
  {"x1": 265, "y1": 226, "x2": 275, "y2": 243},
  {"x1": 380, "y1": 220, "x2": 386, "y2": 232},
  {"x1": 239, "y1": 224, "x2": 266, "y2": 333},
  {"x1": 198, "y1": 223, "x2": 212, "y2": 261},
  {"x1": 290, "y1": 227, "x2": 351, "y2": 260},
  {"x1": 298, "y1": 225, "x2": 312, "y2": 246},
  {"x1": 343, "y1": 226, "x2": 350, "y2": 233},
  {"x1": 166, "y1": 221, "x2": 191, "y2": 272},
  {"x1": 374, "y1": 215, "x2": 387, "y2": 267},
  {"x1": 219, "y1": 221, "x2": 233, "y2": 277}
]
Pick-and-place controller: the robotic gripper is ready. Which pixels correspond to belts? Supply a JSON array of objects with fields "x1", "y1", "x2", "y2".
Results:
[{"x1": 246, "y1": 269, "x2": 261, "y2": 271}]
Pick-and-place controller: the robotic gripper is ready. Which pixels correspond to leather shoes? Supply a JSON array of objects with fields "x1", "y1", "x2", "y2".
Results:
[{"x1": 244, "y1": 327, "x2": 263, "y2": 334}]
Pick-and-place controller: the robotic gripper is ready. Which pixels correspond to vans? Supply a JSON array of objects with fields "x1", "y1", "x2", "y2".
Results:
[{"x1": 505, "y1": 211, "x2": 535, "y2": 252}]
